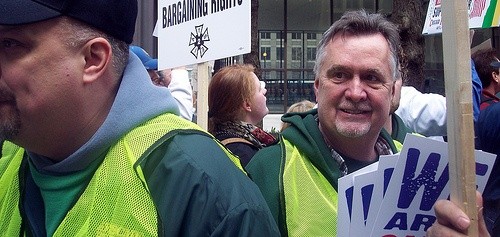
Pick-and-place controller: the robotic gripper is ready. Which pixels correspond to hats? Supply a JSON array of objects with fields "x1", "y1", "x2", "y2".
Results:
[
  {"x1": 128, "y1": 46, "x2": 159, "y2": 71},
  {"x1": 0, "y1": 0, "x2": 139, "y2": 44}
]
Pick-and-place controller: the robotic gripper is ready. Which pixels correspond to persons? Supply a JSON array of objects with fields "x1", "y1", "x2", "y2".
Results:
[
  {"x1": 207, "y1": 64, "x2": 279, "y2": 172},
  {"x1": 281, "y1": 98, "x2": 316, "y2": 131},
  {"x1": 244, "y1": 9, "x2": 424, "y2": 237},
  {"x1": 129, "y1": 46, "x2": 193, "y2": 121},
  {"x1": 0, "y1": 0, "x2": 279, "y2": 237},
  {"x1": 394, "y1": 57, "x2": 483, "y2": 137},
  {"x1": 471, "y1": 52, "x2": 500, "y2": 237},
  {"x1": 426, "y1": 191, "x2": 490, "y2": 237}
]
